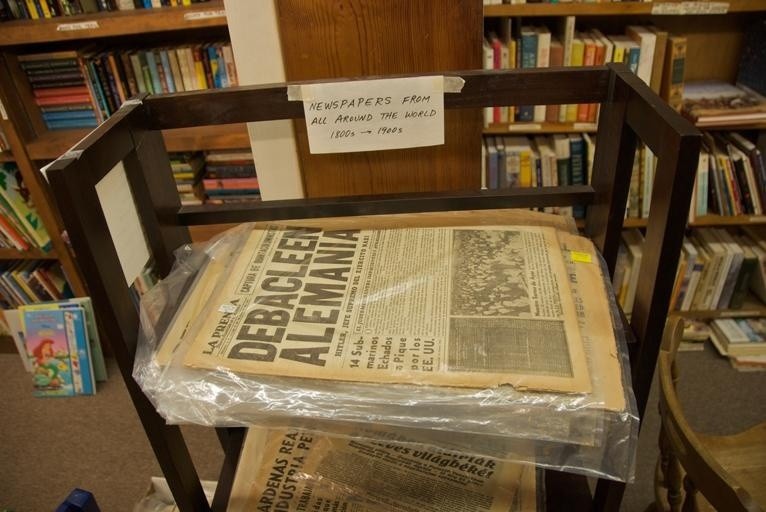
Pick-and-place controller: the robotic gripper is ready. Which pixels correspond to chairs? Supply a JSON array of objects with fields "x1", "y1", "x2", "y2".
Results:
[{"x1": 646, "y1": 313, "x2": 766, "y2": 512}]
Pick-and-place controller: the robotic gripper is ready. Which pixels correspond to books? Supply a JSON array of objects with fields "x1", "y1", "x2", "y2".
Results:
[
  {"x1": 481, "y1": 1, "x2": 765, "y2": 373},
  {"x1": 1, "y1": 1, "x2": 260, "y2": 397}
]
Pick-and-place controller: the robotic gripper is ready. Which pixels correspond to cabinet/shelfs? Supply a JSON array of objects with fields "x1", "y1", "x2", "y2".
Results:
[
  {"x1": 1, "y1": 1, "x2": 260, "y2": 353},
  {"x1": 481, "y1": 0, "x2": 766, "y2": 355},
  {"x1": 47, "y1": 61, "x2": 702, "y2": 511}
]
